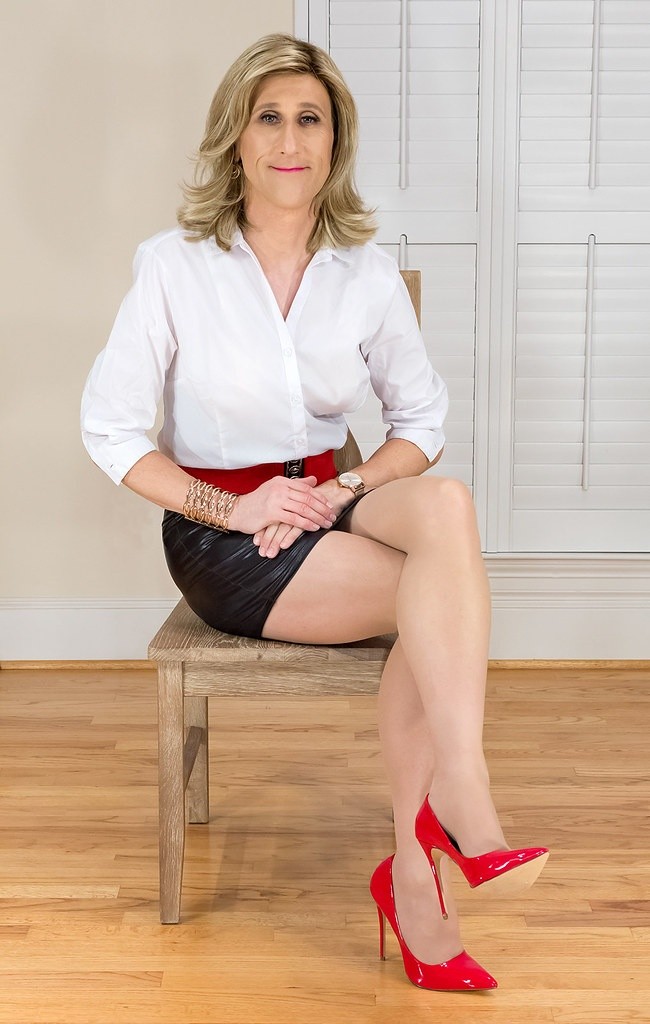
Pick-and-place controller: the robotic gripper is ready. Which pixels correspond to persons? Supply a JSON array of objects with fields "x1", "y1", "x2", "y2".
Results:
[{"x1": 80, "y1": 34, "x2": 550, "y2": 992}]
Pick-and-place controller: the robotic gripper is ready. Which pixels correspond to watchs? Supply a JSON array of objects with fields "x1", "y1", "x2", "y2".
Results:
[{"x1": 333, "y1": 472, "x2": 366, "y2": 497}]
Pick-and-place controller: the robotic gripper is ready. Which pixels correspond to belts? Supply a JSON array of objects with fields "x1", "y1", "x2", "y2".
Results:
[{"x1": 180, "y1": 450, "x2": 339, "y2": 497}]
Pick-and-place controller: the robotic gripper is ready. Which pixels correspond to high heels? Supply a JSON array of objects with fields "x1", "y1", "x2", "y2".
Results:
[
  {"x1": 369, "y1": 854, "x2": 499, "y2": 991},
  {"x1": 416, "y1": 793, "x2": 550, "y2": 919}
]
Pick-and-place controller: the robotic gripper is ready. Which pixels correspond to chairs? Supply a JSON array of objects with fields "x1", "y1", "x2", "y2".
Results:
[{"x1": 139, "y1": 269, "x2": 422, "y2": 929}]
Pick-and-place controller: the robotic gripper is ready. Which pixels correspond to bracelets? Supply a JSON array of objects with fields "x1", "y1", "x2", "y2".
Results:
[{"x1": 183, "y1": 478, "x2": 239, "y2": 534}]
